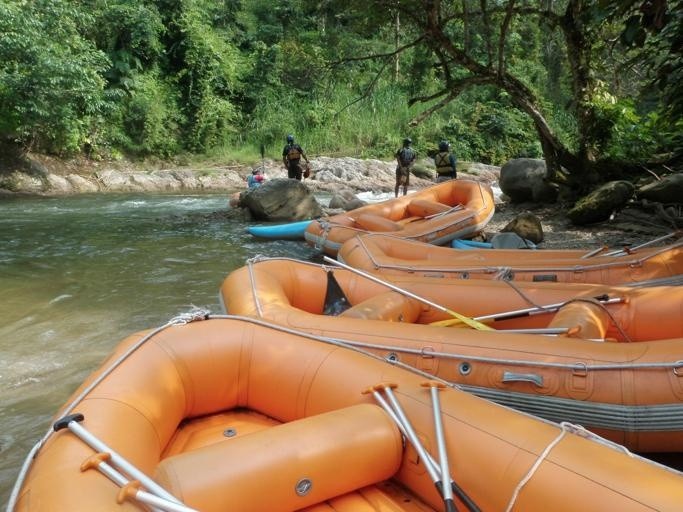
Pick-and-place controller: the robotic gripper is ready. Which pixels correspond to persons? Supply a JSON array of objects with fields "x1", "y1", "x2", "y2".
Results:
[
  {"x1": 247, "y1": 168, "x2": 267, "y2": 189},
  {"x1": 434, "y1": 141, "x2": 456, "y2": 184},
  {"x1": 392, "y1": 138, "x2": 416, "y2": 198},
  {"x1": 281, "y1": 135, "x2": 309, "y2": 182}
]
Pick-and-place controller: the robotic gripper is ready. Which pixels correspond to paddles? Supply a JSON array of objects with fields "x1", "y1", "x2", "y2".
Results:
[
  {"x1": 323, "y1": 256, "x2": 497, "y2": 331},
  {"x1": 429, "y1": 293, "x2": 630, "y2": 329}
]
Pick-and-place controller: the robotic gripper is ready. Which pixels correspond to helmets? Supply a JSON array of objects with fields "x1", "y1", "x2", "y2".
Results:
[
  {"x1": 286, "y1": 134, "x2": 294, "y2": 144},
  {"x1": 438, "y1": 141, "x2": 450, "y2": 151},
  {"x1": 403, "y1": 138, "x2": 412, "y2": 146}
]
